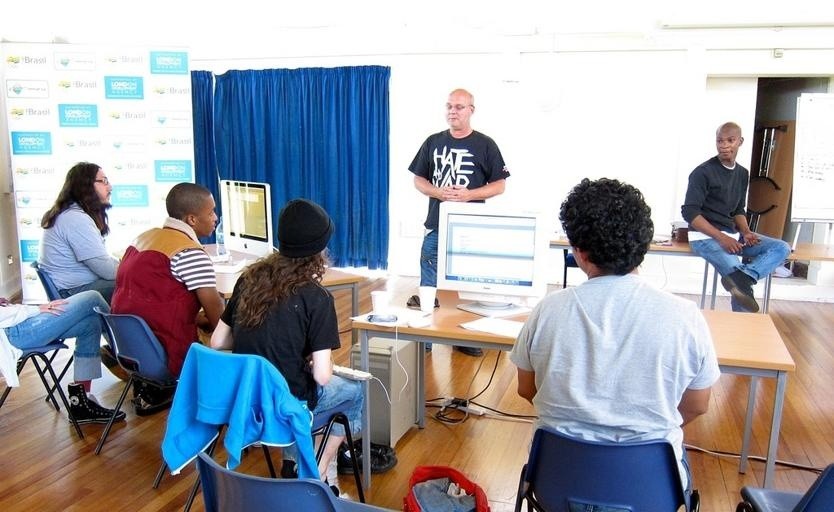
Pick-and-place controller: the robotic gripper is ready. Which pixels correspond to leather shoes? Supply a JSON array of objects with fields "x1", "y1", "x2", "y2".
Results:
[{"x1": 457, "y1": 346, "x2": 484, "y2": 356}]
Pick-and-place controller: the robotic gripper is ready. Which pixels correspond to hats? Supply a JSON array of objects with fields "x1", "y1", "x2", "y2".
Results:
[{"x1": 277, "y1": 198, "x2": 334, "y2": 258}]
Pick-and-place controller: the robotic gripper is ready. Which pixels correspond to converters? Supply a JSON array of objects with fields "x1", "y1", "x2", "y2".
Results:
[{"x1": 452, "y1": 398, "x2": 467, "y2": 407}]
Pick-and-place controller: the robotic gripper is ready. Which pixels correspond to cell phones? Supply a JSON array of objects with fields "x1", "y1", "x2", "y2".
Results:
[{"x1": 368, "y1": 314, "x2": 398, "y2": 323}]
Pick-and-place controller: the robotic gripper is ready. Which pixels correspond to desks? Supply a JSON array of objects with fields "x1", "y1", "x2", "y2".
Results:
[
  {"x1": 199, "y1": 239, "x2": 361, "y2": 365},
  {"x1": 348, "y1": 292, "x2": 798, "y2": 492},
  {"x1": 549, "y1": 224, "x2": 834, "y2": 316},
  {"x1": 750, "y1": 119, "x2": 797, "y2": 244}
]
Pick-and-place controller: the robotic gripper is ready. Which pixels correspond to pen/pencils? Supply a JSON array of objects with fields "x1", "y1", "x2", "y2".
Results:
[{"x1": 655, "y1": 242, "x2": 672, "y2": 246}]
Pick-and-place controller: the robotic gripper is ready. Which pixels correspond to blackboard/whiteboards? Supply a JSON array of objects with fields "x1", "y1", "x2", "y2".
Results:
[{"x1": 790, "y1": 90, "x2": 830, "y2": 224}]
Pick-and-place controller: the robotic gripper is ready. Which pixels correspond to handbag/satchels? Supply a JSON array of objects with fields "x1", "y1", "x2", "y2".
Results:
[{"x1": 404, "y1": 467, "x2": 491, "y2": 512}]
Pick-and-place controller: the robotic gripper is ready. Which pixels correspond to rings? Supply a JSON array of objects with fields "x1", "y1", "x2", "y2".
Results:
[{"x1": 49, "y1": 305, "x2": 51, "y2": 309}]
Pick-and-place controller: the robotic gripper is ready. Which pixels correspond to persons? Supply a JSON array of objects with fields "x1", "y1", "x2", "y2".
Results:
[
  {"x1": 508, "y1": 176, "x2": 724, "y2": 511},
  {"x1": 405, "y1": 87, "x2": 511, "y2": 358},
  {"x1": 37, "y1": 161, "x2": 124, "y2": 306},
  {"x1": 110, "y1": 181, "x2": 226, "y2": 417},
  {"x1": 0, "y1": 288, "x2": 126, "y2": 427},
  {"x1": 680, "y1": 121, "x2": 792, "y2": 314},
  {"x1": 209, "y1": 196, "x2": 366, "y2": 502}
]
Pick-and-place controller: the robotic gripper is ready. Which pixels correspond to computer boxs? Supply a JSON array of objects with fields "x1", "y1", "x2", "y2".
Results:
[{"x1": 350, "y1": 336, "x2": 419, "y2": 449}]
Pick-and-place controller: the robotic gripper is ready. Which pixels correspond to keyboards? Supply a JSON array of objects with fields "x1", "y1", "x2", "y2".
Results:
[{"x1": 332, "y1": 363, "x2": 373, "y2": 382}]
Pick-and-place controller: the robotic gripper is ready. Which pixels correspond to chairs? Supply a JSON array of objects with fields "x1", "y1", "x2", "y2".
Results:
[
  {"x1": 734, "y1": 463, "x2": 834, "y2": 510},
  {"x1": 193, "y1": 452, "x2": 396, "y2": 512},
  {"x1": 515, "y1": 425, "x2": 701, "y2": 512},
  {"x1": 179, "y1": 341, "x2": 367, "y2": 512}
]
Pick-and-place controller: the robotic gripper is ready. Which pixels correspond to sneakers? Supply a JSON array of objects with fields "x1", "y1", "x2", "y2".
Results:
[
  {"x1": 340, "y1": 439, "x2": 394, "y2": 455},
  {"x1": 722, "y1": 269, "x2": 759, "y2": 312},
  {"x1": 100, "y1": 344, "x2": 130, "y2": 381},
  {"x1": 134, "y1": 380, "x2": 177, "y2": 416},
  {"x1": 337, "y1": 448, "x2": 396, "y2": 475}
]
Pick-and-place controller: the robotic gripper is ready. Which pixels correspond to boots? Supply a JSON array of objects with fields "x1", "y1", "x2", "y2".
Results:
[{"x1": 68, "y1": 383, "x2": 125, "y2": 425}]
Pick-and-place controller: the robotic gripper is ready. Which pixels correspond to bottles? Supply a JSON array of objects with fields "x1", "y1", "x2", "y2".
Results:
[{"x1": 215, "y1": 216, "x2": 229, "y2": 256}]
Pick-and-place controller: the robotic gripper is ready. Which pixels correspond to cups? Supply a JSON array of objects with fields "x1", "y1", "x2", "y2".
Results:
[
  {"x1": 370, "y1": 291, "x2": 391, "y2": 318},
  {"x1": 417, "y1": 285, "x2": 437, "y2": 313}
]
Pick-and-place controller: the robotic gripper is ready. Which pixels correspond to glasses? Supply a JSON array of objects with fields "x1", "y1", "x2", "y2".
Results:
[
  {"x1": 406, "y1": 296, "x2": 441, "y2": 307},
  {"x1": 93, "y1": 177, "x2": 109, "y2": 185},
  {"x1": 447, "y1": 105, "x2": 474, "y2": 112}
]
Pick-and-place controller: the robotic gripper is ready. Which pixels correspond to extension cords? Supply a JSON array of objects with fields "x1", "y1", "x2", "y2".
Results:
[{"x1": 444, "y1": 398, "x2": 485, "y2": 416}]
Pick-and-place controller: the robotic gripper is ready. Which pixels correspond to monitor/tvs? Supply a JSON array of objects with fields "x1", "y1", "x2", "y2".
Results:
[
  {"x1": 220, "y1": 179, "x2": 272, "y2": 267},
  {"x1": 436, "y1": 199, "x2": 543, "y2": 318}
]
